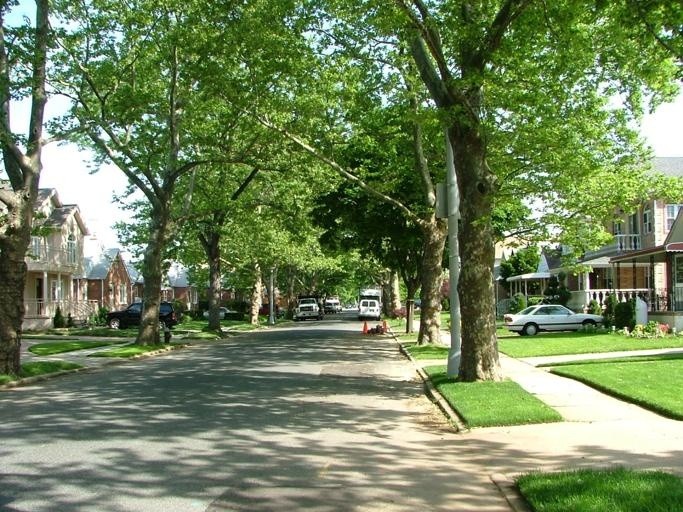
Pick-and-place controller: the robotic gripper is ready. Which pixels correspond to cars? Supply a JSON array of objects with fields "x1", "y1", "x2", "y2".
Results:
[
  {"x1": 259, "y1": 304, "x2": 279, "y2": 315},
  {"x1": 293, "y1": 297, "x2": 323, "y2": 321},
  {"x1": 504, "y1": 304, "x2": 604, "y2": 336},
  {"x1": 202, "y1": 306, "x2": 238, "y2": 319},
  {"x1": 324, "y1": 300, "x2": 342, "y2": 314}
]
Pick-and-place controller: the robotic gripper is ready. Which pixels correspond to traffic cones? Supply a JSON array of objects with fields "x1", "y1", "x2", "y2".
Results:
[{"x1": 363, "y1": 321, "x2": 368, "y2": 336}]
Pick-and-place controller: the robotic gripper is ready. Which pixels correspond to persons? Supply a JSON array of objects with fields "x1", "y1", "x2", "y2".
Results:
[{"x1": 163, "y1": 311, "x2": 179, "y2": 343}]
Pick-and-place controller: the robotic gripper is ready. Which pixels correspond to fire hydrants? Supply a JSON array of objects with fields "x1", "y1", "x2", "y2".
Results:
[{"x1": 164, "y1": 328, "x2": 170, "y2": 344}]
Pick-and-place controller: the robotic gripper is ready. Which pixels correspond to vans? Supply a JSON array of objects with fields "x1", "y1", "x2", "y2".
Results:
[{"x1": 358, "y1": 289, "x2": 381, "y2": 321}]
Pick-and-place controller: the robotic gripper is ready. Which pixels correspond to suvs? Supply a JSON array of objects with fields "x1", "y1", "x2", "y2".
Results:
[{"x1": 106, "y1": 302, "x2": 178, "y2": 329}]
